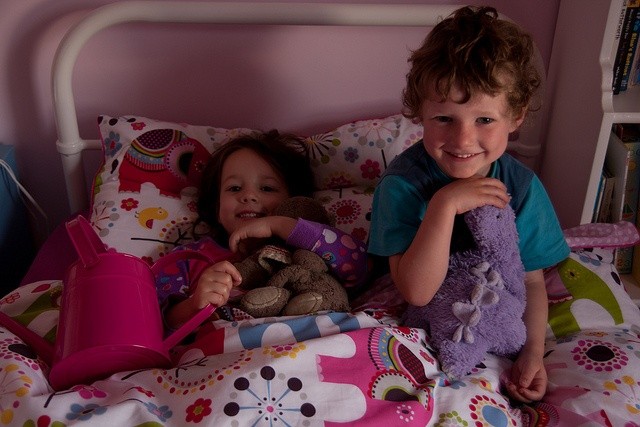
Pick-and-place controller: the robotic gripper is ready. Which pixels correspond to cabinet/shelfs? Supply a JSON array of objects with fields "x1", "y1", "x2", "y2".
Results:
[{"x1": 539, "y1": 0, "x2": 639, "y2": 265}]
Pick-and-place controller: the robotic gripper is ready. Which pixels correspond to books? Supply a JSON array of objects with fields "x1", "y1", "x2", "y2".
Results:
[
  {"x1": 609, "y1": 0, "x2": 640, "y2": 95},
  {"x1": 596, "y1": 125, "x2": 636, "y2": 275}
]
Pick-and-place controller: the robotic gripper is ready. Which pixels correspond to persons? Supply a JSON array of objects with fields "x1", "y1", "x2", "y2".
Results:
[
  {"x1": 153, "y1": 132, "x2": 367, "y2": 345},
  {"x1": 368, "y1": 2, "x2": 571, "y2": 403}
]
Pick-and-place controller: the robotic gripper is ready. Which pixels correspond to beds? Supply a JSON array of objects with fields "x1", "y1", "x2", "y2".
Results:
[{"x1": 2, "y1": 0, "x2": 546, "y2": 426}]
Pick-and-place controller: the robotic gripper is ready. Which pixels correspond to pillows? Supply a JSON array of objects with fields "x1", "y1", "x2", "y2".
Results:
[{"x1": 89, "y1": 110, "x2": 422, "y2": 289}]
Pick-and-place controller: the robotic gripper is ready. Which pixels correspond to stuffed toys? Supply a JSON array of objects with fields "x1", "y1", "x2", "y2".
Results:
[
  {"x1": 232, "y1": 197, "x2": 350, "y2": 316},
  {"x1": 403, "y1": 196, "x2": 528, "y2": 377}
]
